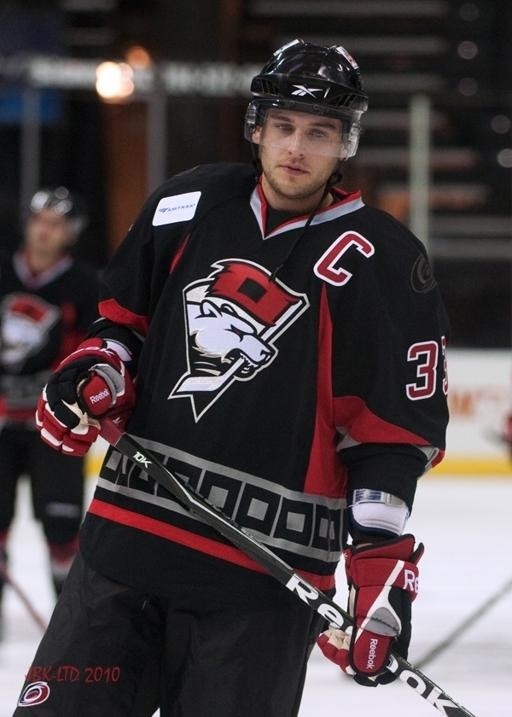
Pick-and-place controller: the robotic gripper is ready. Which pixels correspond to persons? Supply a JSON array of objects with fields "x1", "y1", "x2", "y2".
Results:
[
  {"x1": 7, "y1": 34, "x2": 451, "y2": 715},
  {"x1": 0, "y1": 185, "x2": 115, "y2": 630}
]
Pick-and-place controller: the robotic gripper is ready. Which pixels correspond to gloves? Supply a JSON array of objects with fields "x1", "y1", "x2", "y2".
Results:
[
  {"x1": 35, "y1": 339, "x2": 137, "y2": 458},
  {"x1": 313, "y1": 534, "x2": 425, "y2": 687}
]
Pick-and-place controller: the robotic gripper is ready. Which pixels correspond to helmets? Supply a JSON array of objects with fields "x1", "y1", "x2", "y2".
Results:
[
  {"x1": 244, "y1": 39, "x2": 371, "y2": 160},
  {"x1": 26, "y1": 185, "x2": 88, "y2": 238}
]
